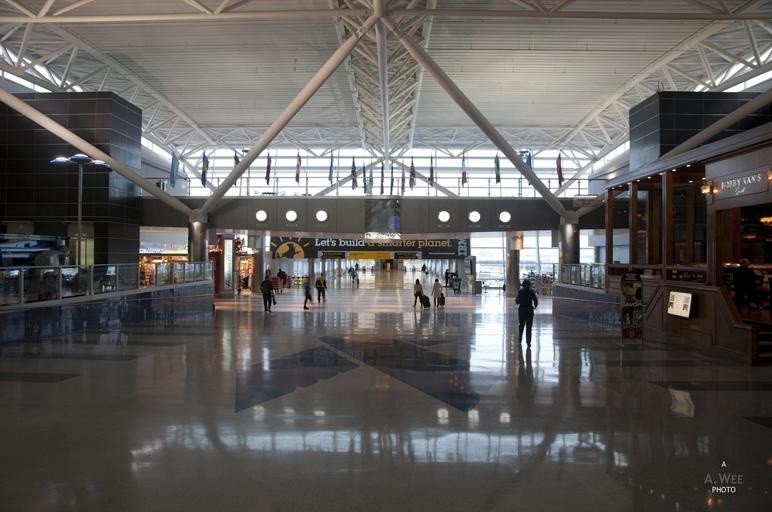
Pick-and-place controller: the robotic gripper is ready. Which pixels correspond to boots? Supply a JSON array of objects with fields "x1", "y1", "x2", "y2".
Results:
[{"x1": 304, "y1": 306, "x2": 309, "y2": 309}]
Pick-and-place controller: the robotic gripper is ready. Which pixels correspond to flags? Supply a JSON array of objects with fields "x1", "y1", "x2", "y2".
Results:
[
  {"x1": 328, "y1": 149, "x2": 334, "y2": 188},
  {"x1": 265, "y1": 153, "x2": 272, "y2": 184},
  {"x1": 557, "y1": 153, "x2": 564, "y2": 187},
  {"x1": 494, "y1": 153, "x2": 501, "y2": 183},
  {"x1": 170, "y1": 151, "x2": 179, "y2": 187},
  {"x1": 526, "y1": 152, "x2": 532, "y2": 186},
  {"x1": 429, "y1": 156, "x2": 434, "y2": 188},
  {"x1": 462, "y1": 151, "x2": 467, "y2": 187},
  {"x1": 234, "y1": 150, "x2": 239, "y2": 186},
  {"x1": 349, "y1": 156, "x2": 416, "y2": 195},
  {"x1": 201, "y1": 154, "x2": 209, "y2": 187},
  {"x1": 296, "y1": 153, "x2": 301, "y2": 183}
]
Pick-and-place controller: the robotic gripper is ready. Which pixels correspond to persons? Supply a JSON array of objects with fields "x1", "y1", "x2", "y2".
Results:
[
  {"x1": 516, "y1": 278, "x2": 538, "y2": 348},
  {"x1": 277, "y1": 268, "x2": 285, "y2": 295},
  {"x1": 517, "y1": 348, "x2": 539, "y2": 407},
  {"x1": 303, "y1": 278, "x2": 312, "y2": 309},
  {"x1": 413, "y1": 279, "x2": 423, "y2": 307},
  {"x1": 422, "y1": 264, "x2": 426, "y2": 273},
  {"x1": 316, "y1": 273, "x2": 327, "y2": 304},
  {"x1": 431, "y1": 279, "x2": 442, "y2": 308},
  {"x1": 260, "y1": 275, "x2": 272, "y2": 313},
  {"x1": 445, "y1": 269, "x2": 451, "y2": 287},
  {"x1": 349, "y1": 263, "x2": 359, "y2": 285},
  {"x1": 733, "y1": 259, "x2": 765, "y2": 303}
]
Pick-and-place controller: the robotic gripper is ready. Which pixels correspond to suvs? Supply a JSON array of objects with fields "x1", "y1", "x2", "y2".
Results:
[{"x1": 0, "y1": 236, "x2": 71, "y2": 301}]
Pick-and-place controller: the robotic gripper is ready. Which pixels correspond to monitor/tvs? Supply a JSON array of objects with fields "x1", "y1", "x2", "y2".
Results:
[
  {"x1": 464, "y1": 258, "x2": 472, "y2": 275},
  {"x1": 667, "y1": 291, "x2": 695, "y2": 320}
]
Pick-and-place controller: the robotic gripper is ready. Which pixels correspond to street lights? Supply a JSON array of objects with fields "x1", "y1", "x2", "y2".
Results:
[{"x1": 51, "y1": 154, "x2": 113, "y2": 292}]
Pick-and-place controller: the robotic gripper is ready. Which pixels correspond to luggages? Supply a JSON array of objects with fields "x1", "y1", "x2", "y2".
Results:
[
  {"x1": 420, "y1": 294, "x2": 430, "y2": 308},
  {"x1": 357, "y1": 274, "x2": 359, "y2": 284},
  {"x1": 440, "y1": 293, "x2": 445, "y2": 305}
]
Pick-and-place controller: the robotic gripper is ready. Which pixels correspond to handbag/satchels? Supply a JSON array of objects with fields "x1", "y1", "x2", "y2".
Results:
[{"x1": 273, "y1": 295, "x2": 276, "y2": 305}]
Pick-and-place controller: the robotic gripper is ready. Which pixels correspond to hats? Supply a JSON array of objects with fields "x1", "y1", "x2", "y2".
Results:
[{"x1": 519, "y1": 279, "x2": 531, "y2": 287}]
